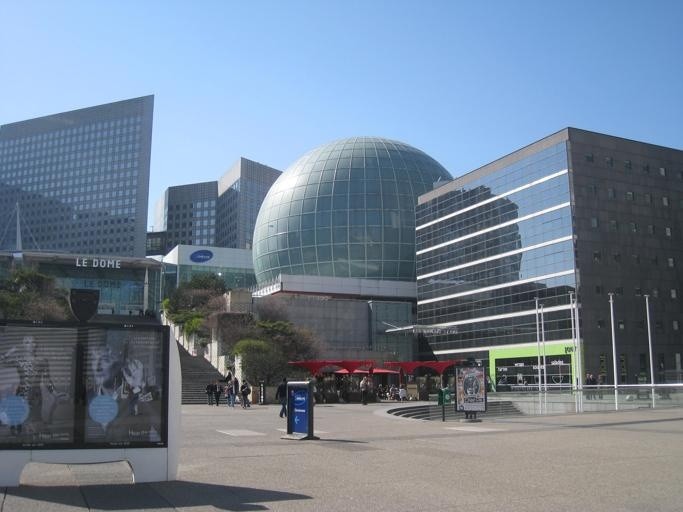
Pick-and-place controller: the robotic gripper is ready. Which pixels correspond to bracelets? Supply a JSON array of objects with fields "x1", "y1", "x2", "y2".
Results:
[{"x1": 132, "y1": 383, "x2": 159, "y2": 403}]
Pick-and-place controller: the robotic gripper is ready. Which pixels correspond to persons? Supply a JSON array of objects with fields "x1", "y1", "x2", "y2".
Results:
[
  {"x1": 275, "y1": 377, "x2": 287, "y2": 417},
  {"x1": 585, "y1": 373, "x2": 604, "y2": 400},
  {"x1": 205, "y1": 371, "x2": 251, "y2": 408},
  {"x1": 305, "y1": 373, "x2": 455, "y2": 406},
  {"x1": 0, "y1": 334, "x2": 70, "y2": 438},
  {"x1": 85, "y1": 330, "x2": 161, "y2": 443}
]
